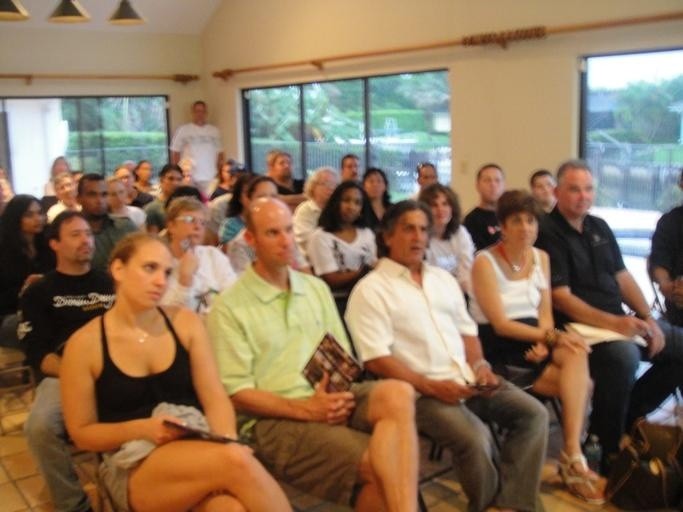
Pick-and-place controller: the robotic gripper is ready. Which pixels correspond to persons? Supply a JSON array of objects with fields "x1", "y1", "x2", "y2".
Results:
[
  {"x1": 167, "y1": 98, "x2": 226, "y2": 193},
  {"x1": 0, "y1": 151, "x2": 682, "y2": 512}
]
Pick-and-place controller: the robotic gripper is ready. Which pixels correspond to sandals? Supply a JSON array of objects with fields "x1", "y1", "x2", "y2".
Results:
[{"x1": 558, "y1": 448, "x2": 609, "y2": 505}]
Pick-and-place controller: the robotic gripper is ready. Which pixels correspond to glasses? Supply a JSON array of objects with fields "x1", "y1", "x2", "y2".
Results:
[{"x1": 174, "y1": 216, "x2": 208, "y2": 227}]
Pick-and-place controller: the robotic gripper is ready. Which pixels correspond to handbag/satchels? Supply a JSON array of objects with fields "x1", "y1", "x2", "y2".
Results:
[{"x1": 607, "y1": 436, "x2": 682, "y2": 511}]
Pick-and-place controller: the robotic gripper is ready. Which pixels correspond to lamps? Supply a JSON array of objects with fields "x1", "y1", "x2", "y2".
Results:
[{"x1": 0, "y1": 0, "x2": 144, "y2": 27}]
